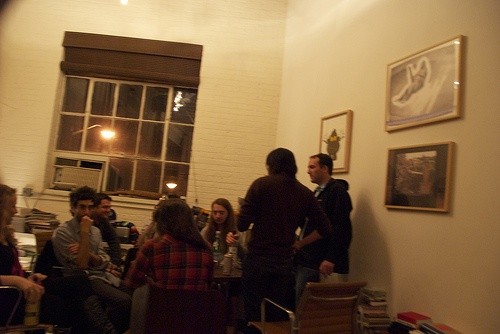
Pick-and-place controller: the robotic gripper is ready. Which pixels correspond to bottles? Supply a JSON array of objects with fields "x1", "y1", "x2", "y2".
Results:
[{"x1": 212, "y1": 230, "x2": 223, "y2": 263}]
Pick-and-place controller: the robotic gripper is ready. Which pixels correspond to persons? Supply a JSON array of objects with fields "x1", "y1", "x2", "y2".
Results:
[
  {"x1": 199, "y1": 198, "x2": 243, "y2": 305},
  {"x1": 235, "y1": 148, "x2": 333, "y2": 334},
  {"x1": 0, "y1": 184, "x2": 48, "y2": 303},
  {"x1": 295, "y1": 153, "x2": 353, "y2": 308},
  {"x1": 51, "y1": 185, "x2": 139, "y2": 334},
  {"x1": 124, "y1": 194, "x2": 215, "y2": 292}
]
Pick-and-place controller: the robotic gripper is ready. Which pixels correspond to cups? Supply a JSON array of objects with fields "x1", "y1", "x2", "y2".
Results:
[
  {"x1": 24, "y1": 292, "x2": 39, "y2": 326},
  {"x1": 228, "y1": 246, "x2": 238, "y2": 267},
  {"x1": 222, "y1": 254, "x2": 232, "y2": 276}
]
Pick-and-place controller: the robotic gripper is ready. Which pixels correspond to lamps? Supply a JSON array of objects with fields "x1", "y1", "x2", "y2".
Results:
[{"x1": 165, "y1": 176, "x2": 177, "y2": 189}]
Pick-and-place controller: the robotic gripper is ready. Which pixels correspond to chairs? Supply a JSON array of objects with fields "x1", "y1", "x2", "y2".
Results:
[{"x1": 247, "y1": 279, "x2": 368, "y2": 334}]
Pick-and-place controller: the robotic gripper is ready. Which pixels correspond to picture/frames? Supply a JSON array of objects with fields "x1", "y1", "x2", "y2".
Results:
[
  {"x1": 384, "y1": 140, "x2": 453, "y2": 213},
  {"x1": 384, "y1": 35, "x2": 464, "y2": 133},
  {"x1": 319, "y1": 110, "x2": 352, "y2": 174}
]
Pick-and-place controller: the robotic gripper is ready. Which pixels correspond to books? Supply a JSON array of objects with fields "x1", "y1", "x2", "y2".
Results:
[
  {"x1": 10, "y1": 208, "x2": 60, "y2": 270},
  {"x1": 354, "y1": 288, "x2": 461, "y2": 334}
]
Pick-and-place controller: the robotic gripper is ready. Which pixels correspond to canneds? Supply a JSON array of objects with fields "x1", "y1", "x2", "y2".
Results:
[{"x1": 223, "y1": 254, "x2": 233, "y2": 276}]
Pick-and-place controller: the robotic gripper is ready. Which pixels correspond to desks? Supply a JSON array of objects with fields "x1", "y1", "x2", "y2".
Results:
[{"x1": 211, "y1": 267, "x2": 242, "y2": 334}]
